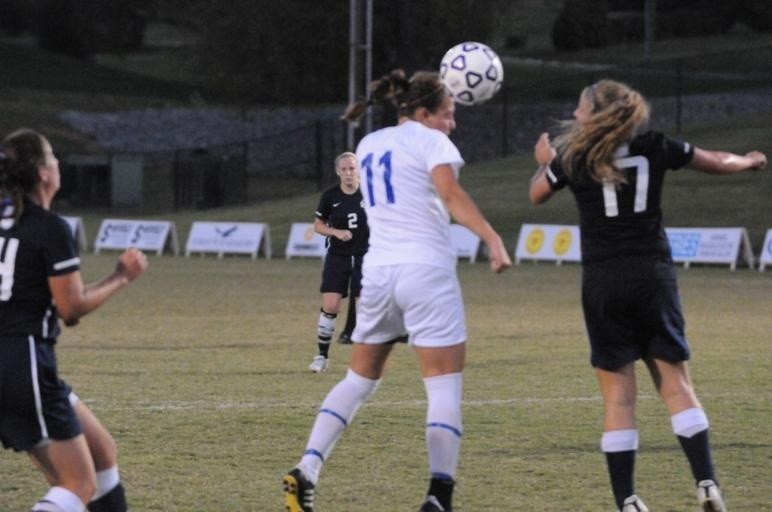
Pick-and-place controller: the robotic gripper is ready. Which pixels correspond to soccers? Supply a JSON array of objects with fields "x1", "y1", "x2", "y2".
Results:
[{"x1": 438, "y1": 41, "x2": 504, "y2": 106}]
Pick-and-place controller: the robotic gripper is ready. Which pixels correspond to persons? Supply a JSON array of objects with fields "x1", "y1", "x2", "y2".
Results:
[
  {"x1": 527, "y1": 78, "x2": 767, "y2": 512},
  {"x1": 283, "y1": 69, "x2": 514, "y2": 512},
  {"x1": 0, "y1": 129, "x2": 150, "y2": 512},
  {"x1": 307, "y1": 151, "x2": 365, "y2": 373}
]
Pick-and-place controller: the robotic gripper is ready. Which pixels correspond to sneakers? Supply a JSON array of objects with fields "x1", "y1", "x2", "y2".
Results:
[
  {"x1": 282, "y1": 468, "x2": 314, "y2": 512},
  {"x1": 416, "y1": 496, "x2": 445, "y2": 512},
  {"x1": 308, "y1": 356, "x2": 329, "y2": 373},
  {"x1": 621, "y1": 494, "x2": 649, "y2": 512},
  {"x1": 696, "y1": 479, "x2": 727, "y2": 512}
]
